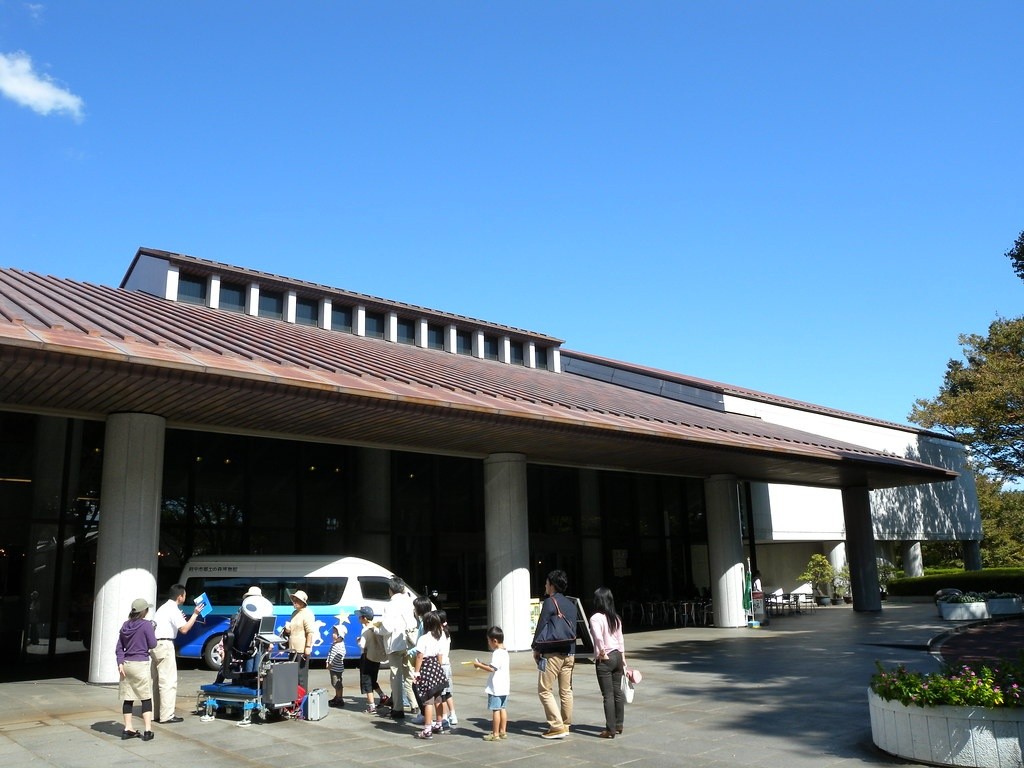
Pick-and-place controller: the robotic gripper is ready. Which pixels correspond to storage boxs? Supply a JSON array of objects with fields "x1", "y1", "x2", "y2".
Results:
[{"x1": 308, "y1": 687, "x2": 330, "y2": 721}]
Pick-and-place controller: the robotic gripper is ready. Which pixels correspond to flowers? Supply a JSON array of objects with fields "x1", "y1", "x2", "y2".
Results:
[
  {"x1": 939, "y1": 589, "x2": 1019, "y2": 603},
  {"x1": 869, "y1": 654, "x2": 1024, "y2": 710}
]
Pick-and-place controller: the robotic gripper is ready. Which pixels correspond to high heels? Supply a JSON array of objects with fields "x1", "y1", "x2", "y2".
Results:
[
  {"x1": 600, "y1": 731, "x2": 615, "y2": 739},
  {"x1": 616, "y1": 728, "x2": 622, "y2": 734}
]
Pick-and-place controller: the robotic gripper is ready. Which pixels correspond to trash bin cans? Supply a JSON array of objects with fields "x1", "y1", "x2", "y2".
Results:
[{"x1": 752, "y1": 592, "x2": 769, "y2": 626}]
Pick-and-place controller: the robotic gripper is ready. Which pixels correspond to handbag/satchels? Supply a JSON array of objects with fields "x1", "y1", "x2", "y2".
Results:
[
  {"x1": 534, "y1": 596, "x2": 577, "y2": 646},
  {"x1": 620, "y1": 675, "x2": 635, "y2": 703}
]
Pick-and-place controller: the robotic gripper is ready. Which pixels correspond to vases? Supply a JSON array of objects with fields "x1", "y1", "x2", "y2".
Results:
[
  {"x1": 935, "y1": 597, "x2": 1022, "y2": 622},
  {"x1": 868, "y1": 685, "x2": 1024, "y2": 768}
]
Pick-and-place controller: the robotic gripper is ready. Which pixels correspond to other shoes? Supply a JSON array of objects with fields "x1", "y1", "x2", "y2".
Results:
[
  {"x1": 377, "y1": 695, "x2": 389, "y2": 708},
  {"x1": 385, "y1": 710, "x2": 404, "y2": 718},
  {"x1": 432, "y1": 726, "x2": 444, "y2": 734},
  {"x1": 416, "y1": 731, "x2": 433, "y2": 739},
  {"x1": 411, "y1": 708, "x2": 419, "y2": 714},
  {"x1": 442, "y1": 720, "x2": 449, "y2": 728},
  {"x1": 541, "y1": 729, "x2": 565, "y2": 739},
  {"x1": 328, "y1": 699, "x2": 344, "y2": 707},
  {"x1": 565, "y1": 724, "x2": 570, "y2": 736},
  {"x1": 361, "y1": 704, "x2": 377, "y2": 714},
  {"x1": 449, "y1": 716, "x2": 458, "y2": 724}
]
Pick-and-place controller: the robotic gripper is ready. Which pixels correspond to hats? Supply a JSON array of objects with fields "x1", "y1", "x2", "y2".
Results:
[
  {"x1": 290, "y1": 590, "x2": 308, "y2": 605},
  {"x1": 131, "y1": 599, "x2": 153, "y2": 614},
  {"x1": 333, "y1": 624, "x2": 347, "y2": 639},
  {"x1": 354, "y1": 606, "x2": 373, "y2": 620},
  {"x1": 242, "y1": 586, "x2": 262, "y2": 596}
]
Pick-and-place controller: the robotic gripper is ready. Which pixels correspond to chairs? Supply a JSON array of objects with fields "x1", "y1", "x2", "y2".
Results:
[{"x1": 622, "y1": 593, "x2": 817, "y2": 629}]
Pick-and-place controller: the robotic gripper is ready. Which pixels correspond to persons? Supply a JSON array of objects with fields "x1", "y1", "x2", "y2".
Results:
[
  {"x1": 410, "y1": 595, "x2": 458, "y2": 739},
  {"x1": 282, "y1": 589, "x2": 316, "y2": 694},
  {"x1": 355, "y1": 606, "x2": 390, "y2": 714},
  {"x1": 752, "y1": 569, "x2": 763, "y2": 625},
  {"x1": 30, "y1": 593, "x2": 39, "y2": 644},
  {"x1": 326, "y1": 624, "x2": 348, "y2": 708},
  {"x1": 532, "y1": 570, "x2": 578, "y2": 739},
  {"x1": 218, "y1": 586, "x2": 264, "y2": 673},
  {"x1": 474, "y1": 626, "x2": 511, "y2": 741},
  {"x1": 148, "y1": 584, "x2": 204, "y2": 723},
  {"x1": 116, "y1": 599, "x2": 158, "y2": 741},
  {"x1": 369, "y1": 576, "x2": 421, "y2": 721},
  {"x1": 589, "y1": 587, "x2": 627, "y2": 738}
]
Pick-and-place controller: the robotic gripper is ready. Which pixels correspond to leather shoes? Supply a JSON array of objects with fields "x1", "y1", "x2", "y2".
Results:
[
  {"x1": 167, "y1": 715, "x2": 183, "y2": 723},
  {"x1": 122, "y1": 730, "x2": 140, "y2": 740},
  {"x1": 144, "y1": 731, "x2": 154, "y2": 741}
]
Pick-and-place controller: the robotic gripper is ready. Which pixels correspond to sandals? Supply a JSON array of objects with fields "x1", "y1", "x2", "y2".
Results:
[
  {"x1": 499, "y1": 732, "x2": 507, "y2": 739},
  {"x1": 483, "y1": 733, "x2": 500, "y2": 741}
]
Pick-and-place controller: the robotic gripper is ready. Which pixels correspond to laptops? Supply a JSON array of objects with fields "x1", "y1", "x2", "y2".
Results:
[{"x1": 257, "y1": 616, "x2": 287, "y2": 642}]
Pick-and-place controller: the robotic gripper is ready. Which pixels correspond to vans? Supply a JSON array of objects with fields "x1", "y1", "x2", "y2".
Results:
[{"x1": 171, "y1": 553, "x2": 445, "y2": 672}]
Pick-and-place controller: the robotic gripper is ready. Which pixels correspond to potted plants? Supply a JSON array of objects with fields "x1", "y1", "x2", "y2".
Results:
[{"x1": 795, "y1": 553, "x2": 890, "y2": 605}]
